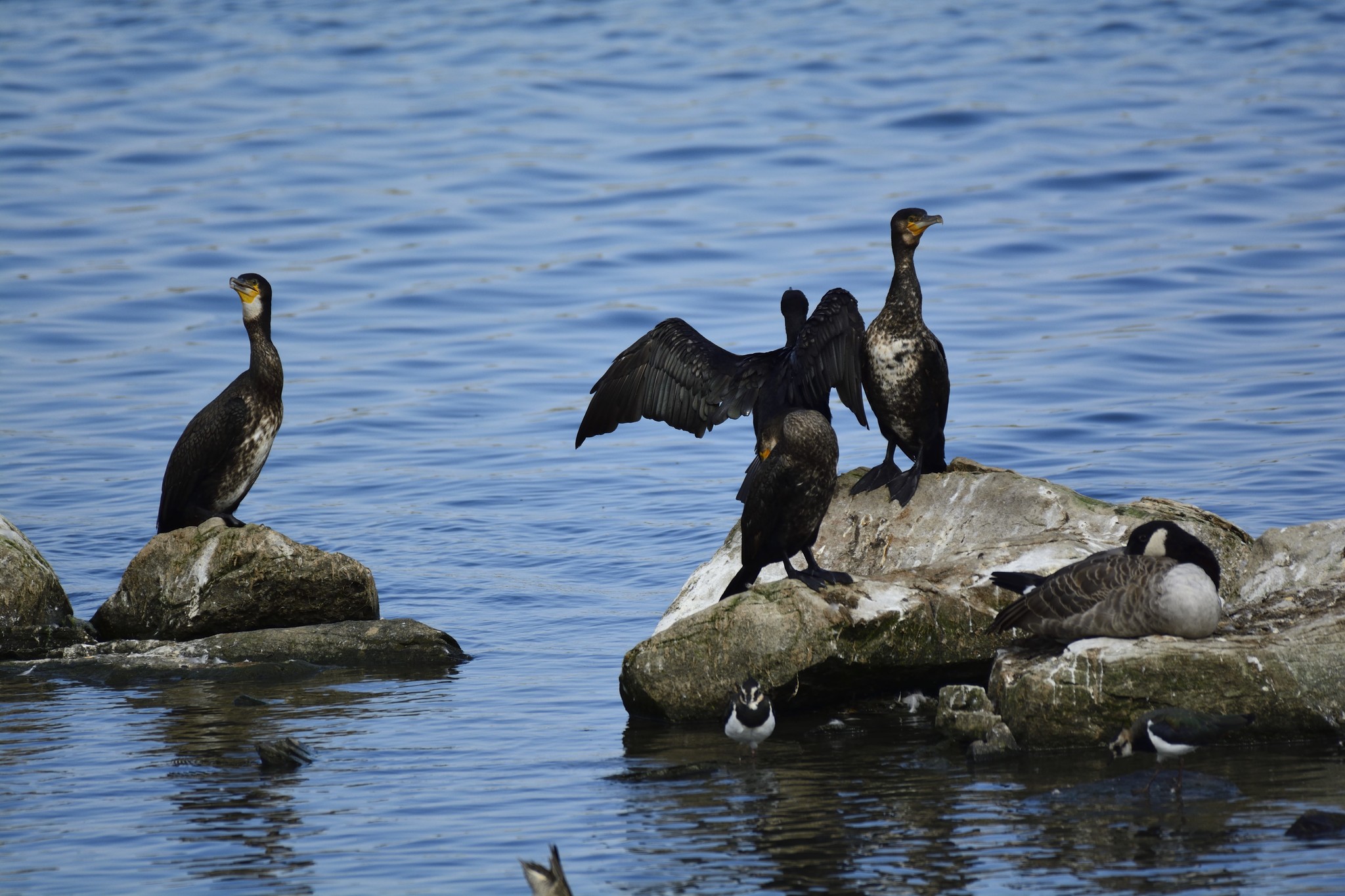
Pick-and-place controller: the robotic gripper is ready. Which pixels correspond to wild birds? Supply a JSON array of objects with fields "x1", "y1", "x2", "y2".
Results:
[
  {"x1": 722, "y1": 679, "x2": 776, "y2": 760},
  {"x1": 156, "y1": 274, "x2": 284, "y2": 529},
  {"x1": 848, "y1": 207, "x2": 950, "y2": 509},
  {"x1": 985, "y1": 521, "x2": 1223, "y2": 645},
  {"x1": 1146, "y1": 712, "x2": 1215, "y2": 794},
  {"x1": 576, "y1": 287, "x2": 869, "y2": 602}
]
